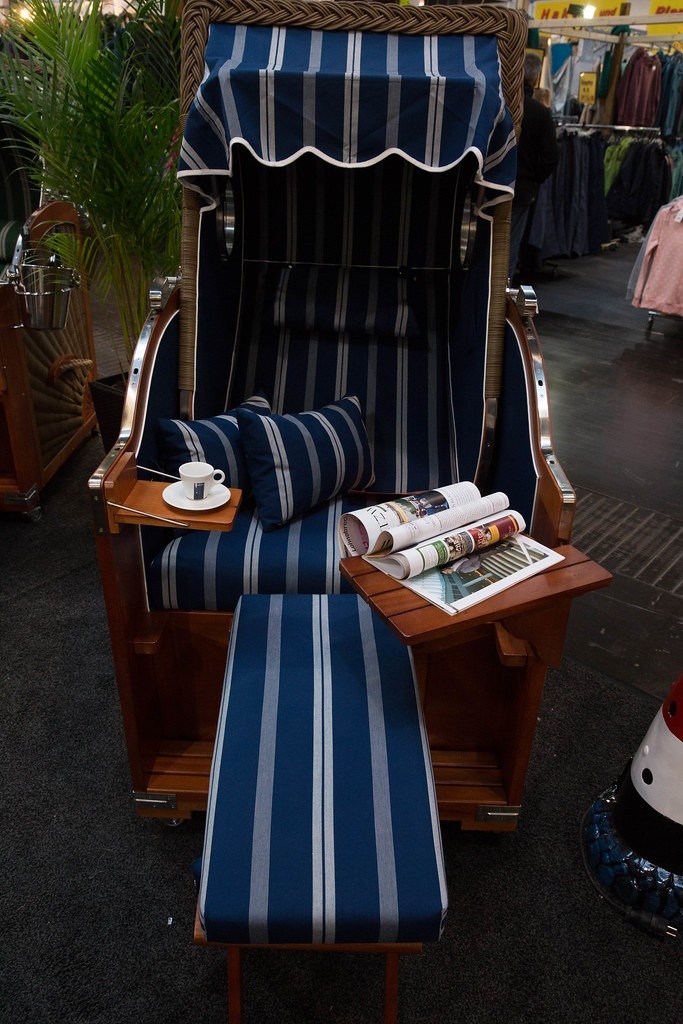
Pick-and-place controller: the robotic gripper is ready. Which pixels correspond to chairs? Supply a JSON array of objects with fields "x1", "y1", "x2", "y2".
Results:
[
  {"x1": 89, "y1": 0, "x2": 612, "y2": 1023},
  {"x1": 0, "y1": 96, "x2": 98, "y2": 522}
]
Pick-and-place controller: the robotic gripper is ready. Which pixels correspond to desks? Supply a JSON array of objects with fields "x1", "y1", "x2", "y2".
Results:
[
  {"x1": 106, "y1": 452, "x2": 246, "y2": 534},
  {"x1": 338, "y1": 552, "x2": 611, "y2": 639}
]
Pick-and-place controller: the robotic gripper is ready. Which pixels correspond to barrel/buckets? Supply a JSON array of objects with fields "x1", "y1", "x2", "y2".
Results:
[{"x1": 7, "y1": 263, "x2": 81, "y2": 330}]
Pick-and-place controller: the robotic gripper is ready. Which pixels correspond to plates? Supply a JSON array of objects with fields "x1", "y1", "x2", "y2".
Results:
[{"x1": 162, "y1": 481, "x2": 231, "y2": 511}]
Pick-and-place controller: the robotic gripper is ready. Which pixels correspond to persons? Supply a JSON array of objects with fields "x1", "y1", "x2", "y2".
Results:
[
  {"x1": 508, "y1": 52, "x2": 560, "y2": 289},
  {"x1": 440, "y1": 543, "x2": 512, "y2": 575}
]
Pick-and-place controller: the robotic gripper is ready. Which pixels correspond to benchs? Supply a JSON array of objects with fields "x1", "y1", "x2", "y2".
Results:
[{"x1": 194, "y1": 594, "x2": 449, "y2": 1023}]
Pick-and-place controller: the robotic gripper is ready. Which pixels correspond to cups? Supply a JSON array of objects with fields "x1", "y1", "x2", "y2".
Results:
[{"x1": 179, "y1": 462, "x2": 225, "y2": 500}]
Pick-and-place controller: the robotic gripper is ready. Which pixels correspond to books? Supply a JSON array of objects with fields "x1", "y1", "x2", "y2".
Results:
[{"x1": 338, "y1": 481, "x2": 564, "y2": 615}]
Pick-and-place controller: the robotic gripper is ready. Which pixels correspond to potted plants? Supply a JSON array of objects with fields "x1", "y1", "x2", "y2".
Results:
[{"x1": 0, "y1": 0, "x2": 181, "y2": 457}]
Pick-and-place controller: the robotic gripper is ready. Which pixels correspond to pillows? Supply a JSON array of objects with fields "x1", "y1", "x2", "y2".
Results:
[
  {"x1": 235, "y1": 394, "x2": 377, "y2": 536},
  {"x1": 152, "y1": 395, "x2": 274, "y2": 488}
]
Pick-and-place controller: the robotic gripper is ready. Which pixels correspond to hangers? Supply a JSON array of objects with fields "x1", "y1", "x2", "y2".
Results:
[
  {"x1": 643, "y1": 39, "x2": 681, "y2": 56},
  {"x1": 606, "y1": 128, "x2": 662, "y2": 146}
]
